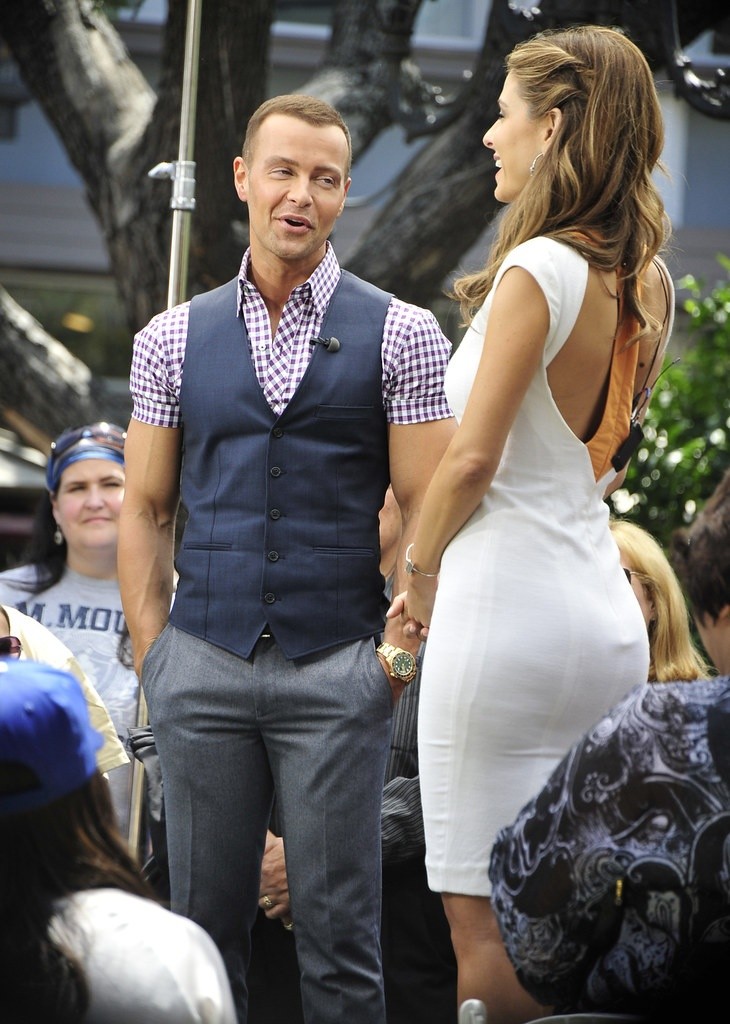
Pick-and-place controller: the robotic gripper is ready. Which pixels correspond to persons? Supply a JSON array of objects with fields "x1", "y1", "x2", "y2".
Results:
[
  {"x1": 491, "y1": 462, "x2": 728, "y2": 1022},
  {"x1": 386, "y1": 27, "x2": 676, "y2": 1024},
  {"x1": 251, "y1": 483, "x2": 451, "y2": 1023},
  {"x1": 0, "y1": 606, "x2": 131, "y2": 776},
  {"x1": 118, "y1": 92, "x2": 459, "y2": 1024},
  {"x1": 0, "y1": 424, "x2": 178, "y2": 902},
  {"x1": 607, "y1": 518, "x2": 705, "y2": 680},
  {"x1": 0, "y1": 658, "x2": 234, "y2": 1024}
]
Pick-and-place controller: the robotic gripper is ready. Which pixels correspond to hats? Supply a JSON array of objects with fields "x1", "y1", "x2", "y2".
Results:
[{"x1": 0, "y1": 653, "x2": 106, "y2": 812}]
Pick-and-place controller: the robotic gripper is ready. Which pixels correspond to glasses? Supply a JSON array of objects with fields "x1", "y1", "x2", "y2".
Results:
[{"x1": 624, "y1": 568, "x2": 647, "y2": 584}]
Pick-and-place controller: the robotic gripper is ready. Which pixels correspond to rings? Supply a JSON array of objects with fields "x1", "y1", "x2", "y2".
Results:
[
  {"x1": 264, "y1": 896, "x2": 273, "y2": 909},
  {"x1": 284, "y1": 922, "x2": 294, "y2": 930}
]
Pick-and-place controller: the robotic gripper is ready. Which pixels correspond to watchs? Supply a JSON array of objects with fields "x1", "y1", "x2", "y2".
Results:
[{"x1": 376, "y1": 642, "x2": 418, "y2": 683}]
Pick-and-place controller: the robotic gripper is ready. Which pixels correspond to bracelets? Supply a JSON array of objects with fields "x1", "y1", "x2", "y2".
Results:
[{"x1": 405, "y1": 543, "x2": 438, "y2": 577}]
